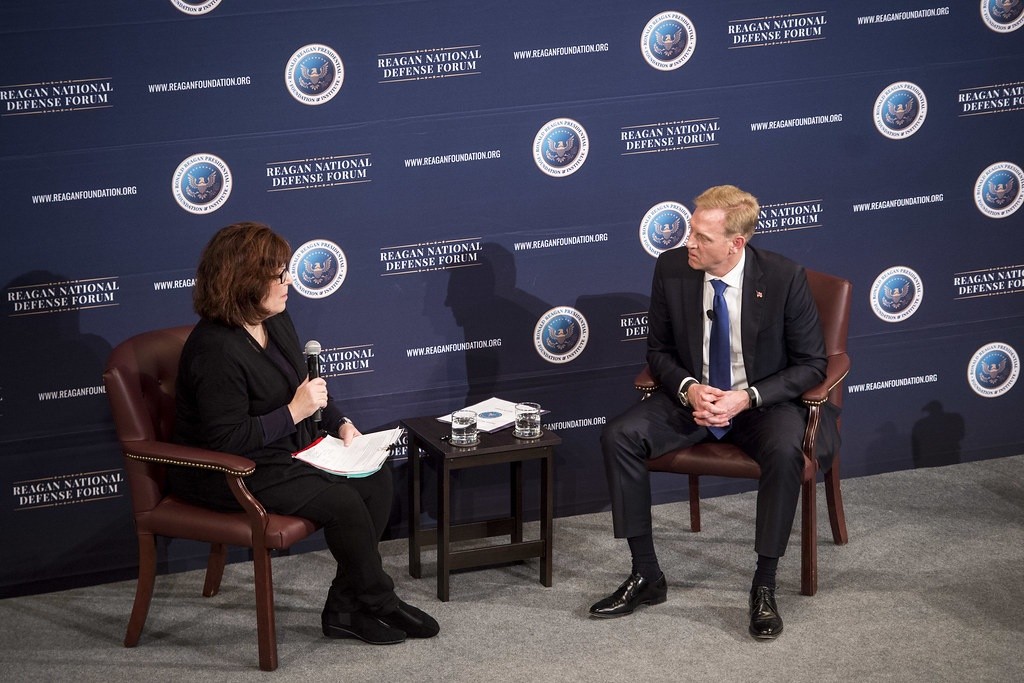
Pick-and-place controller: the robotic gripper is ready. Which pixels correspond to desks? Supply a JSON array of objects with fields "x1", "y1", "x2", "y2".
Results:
[{"x1": 401, "y1": 415, "x2": 561, "y2": 602}]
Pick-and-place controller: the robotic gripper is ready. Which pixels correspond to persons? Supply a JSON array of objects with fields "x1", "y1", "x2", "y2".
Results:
[
  {"x1": 172, "y1": 221, "x2": 440, "y2": 647},
  {"x1": 587, "y1": 185, "x2": 828, "y2": 640}
]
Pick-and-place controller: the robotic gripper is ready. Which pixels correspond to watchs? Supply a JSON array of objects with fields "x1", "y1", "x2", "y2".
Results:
[
  {"x1": 334, "y1": 416, "x2": 355, "y2": 439},
  {"x1": 678, "y1": 380, "x2": 700, "y2": 407},
  {"x1": 742, "y1": 387, "x2": 757, "y2": 409}
]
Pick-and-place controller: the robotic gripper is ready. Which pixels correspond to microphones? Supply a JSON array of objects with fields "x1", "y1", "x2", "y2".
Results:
[
  {"x1": 303, "y1": 340, "x2": 322, "y2": 422},
  {"x1": 706, "y1": 309, "x2": 716, "y2": 320}
]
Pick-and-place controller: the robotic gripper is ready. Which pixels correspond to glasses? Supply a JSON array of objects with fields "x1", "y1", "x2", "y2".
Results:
[{"x1": 269, "y1": 267, "x2": 287, "y2": 284}]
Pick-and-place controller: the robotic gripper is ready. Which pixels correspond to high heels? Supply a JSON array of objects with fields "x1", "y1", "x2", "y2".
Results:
[
  {"x1": 321, "y1": 603, "x2": 407, "y2": 644},
  {"x1": 376, "y1": 593, "x2": 441, "y2": 639}
]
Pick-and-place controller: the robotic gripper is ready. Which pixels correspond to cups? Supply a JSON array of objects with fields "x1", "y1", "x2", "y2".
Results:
[
  {"x1": 515, "y1": 402, "x2": 541, "y2": 437},
  {"x1": 451, "y1": 410, "x2": 477, "y2": 445}
]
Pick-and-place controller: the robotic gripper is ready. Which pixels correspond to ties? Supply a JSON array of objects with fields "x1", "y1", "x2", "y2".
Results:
[{"x1": 708, "y1": 280, "x2": 733, "y2": 439}]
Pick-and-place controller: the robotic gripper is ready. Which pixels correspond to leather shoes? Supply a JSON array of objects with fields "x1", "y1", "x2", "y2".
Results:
[
  {"x1": 589, "y1": 571, "x2": 667, "y2": 618},
  {"x1": 750, "y1": 586, "x2": 784, "y2": 639}
]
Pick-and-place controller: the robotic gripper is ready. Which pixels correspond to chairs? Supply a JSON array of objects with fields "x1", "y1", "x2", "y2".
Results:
[
  {"x1": 104, "y1": 326, "x2": 319, "y2": 672},
  {"x1": 633, "y1": 269, "x2": 852, "y2": 595}
]
